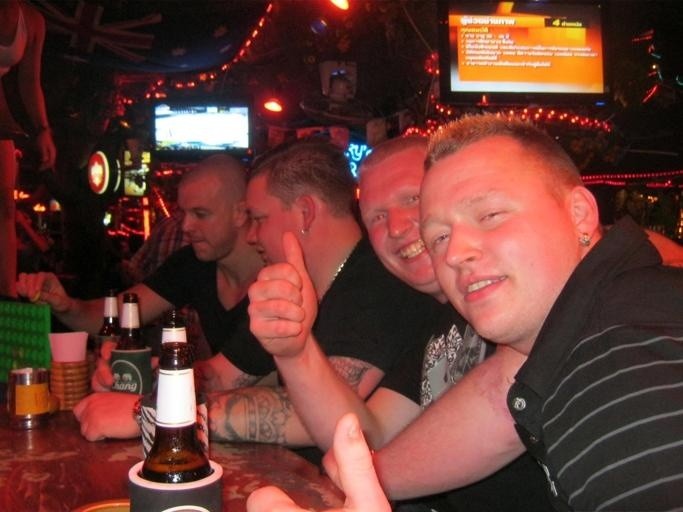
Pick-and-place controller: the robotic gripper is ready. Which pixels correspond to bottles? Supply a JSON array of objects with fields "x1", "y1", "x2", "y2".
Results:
[
  {"x1": 142, "y1": 308, "x2": 214, "y2": 482},
  {"x1": 116, "y1": 292, "x2": 145, "y2": 348},
  {"x1": 98, "y1": 293, "x2": 118, "y2": 330}
]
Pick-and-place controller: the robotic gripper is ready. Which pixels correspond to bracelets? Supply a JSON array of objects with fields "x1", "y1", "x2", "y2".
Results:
[
  {"x1": 131, "y1": 395, "x2": 146, "y2": 436},
  {"x1": 34, "y1": 125, "x2": 47, "y2": 137}
]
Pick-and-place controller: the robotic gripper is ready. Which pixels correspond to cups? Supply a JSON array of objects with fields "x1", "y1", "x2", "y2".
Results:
[{"x1": 11, "y1": 369, "x2": 52, "y2": 429}]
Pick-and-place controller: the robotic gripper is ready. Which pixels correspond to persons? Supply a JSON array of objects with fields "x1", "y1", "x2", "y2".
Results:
[
  {"x1": 12, "y1": 154, "x2": 269, "y2": 357},
  {"x1": 116, "y1": 189, "x2": 192, "y2": 288},
  {"x1": 417, "y1": 109, "x2": 682, "y2": 509},
  {"x1": 0, "y1": 0, "x2": 58, "y2": 302},
  {"x1": 69, "y1": 134, "x2": 445, "y2": 473},
  {"x1": 243, "y1": 130, "x2": 554, "y2": 511}
]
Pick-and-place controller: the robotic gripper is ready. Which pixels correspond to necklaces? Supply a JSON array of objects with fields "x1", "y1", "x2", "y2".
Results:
[{"x1": 328, "y1": 249, "x2": 353, "y2": 287}]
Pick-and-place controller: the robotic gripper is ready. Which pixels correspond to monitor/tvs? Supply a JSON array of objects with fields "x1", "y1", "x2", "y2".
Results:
[
  {"x1": 120, "y1": 148, "x2": 153, "y2": 198},
  {"x1": 148, "y1": 91, "x2": 255, "y2": 163},
  {"x1": 435, "y1": 0, "x2": 610, "y2": 107}
]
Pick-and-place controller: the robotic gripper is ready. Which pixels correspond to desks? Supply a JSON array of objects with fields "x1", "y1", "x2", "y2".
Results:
[{"x1": 0, "y1": 406, "x2": 347, "y2": 511}]
